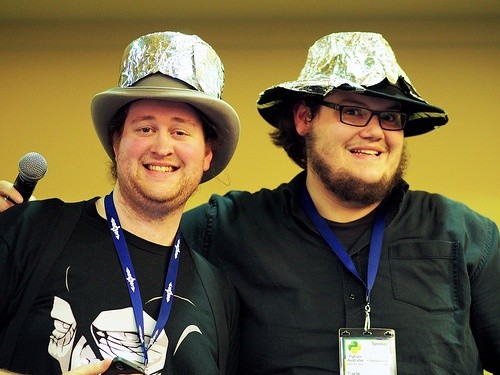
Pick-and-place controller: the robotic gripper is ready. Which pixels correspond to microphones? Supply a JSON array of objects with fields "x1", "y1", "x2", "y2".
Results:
[{"x1": 6, "y1": 152, "x2": 48, "y2": 205}]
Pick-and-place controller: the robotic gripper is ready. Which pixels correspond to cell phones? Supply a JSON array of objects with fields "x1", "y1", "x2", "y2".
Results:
[{"x1": 99, "y1": 357, "x2": 148, "y2": 375}]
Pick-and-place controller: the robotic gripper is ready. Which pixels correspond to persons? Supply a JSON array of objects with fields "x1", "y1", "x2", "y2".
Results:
[
  {"x1": 0, "y1": 32, "x2": 245, "y2": 375},
  {"x1": 0, "y1": 30, "x2": 499, "y2": 375}
]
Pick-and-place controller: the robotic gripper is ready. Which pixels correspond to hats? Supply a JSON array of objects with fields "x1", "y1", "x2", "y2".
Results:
[
  {"x1": 90, "y1": 31, "x2": 241, "y2": 183},
  {"x1": 256, "y1": 31, "x2": 449, "y2": 138}
]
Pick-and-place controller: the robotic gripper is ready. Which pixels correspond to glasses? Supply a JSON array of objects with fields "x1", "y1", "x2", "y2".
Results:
[{"x1": 309, "y1": 97, "x2": 408, "y2": 131}]
